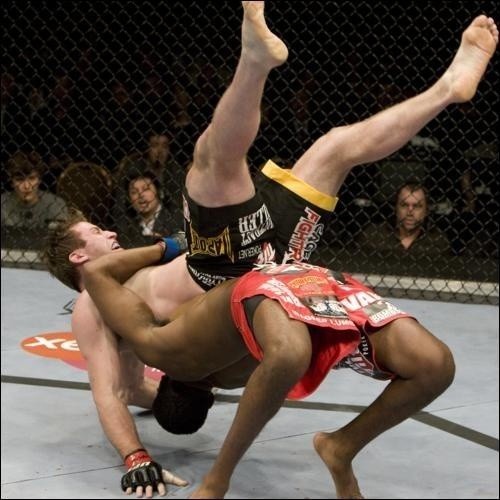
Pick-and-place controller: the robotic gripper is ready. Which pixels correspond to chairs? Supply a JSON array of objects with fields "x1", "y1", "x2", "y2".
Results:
[{"x1": 56, "y1": 161, "x2": 111, "y2": 216}]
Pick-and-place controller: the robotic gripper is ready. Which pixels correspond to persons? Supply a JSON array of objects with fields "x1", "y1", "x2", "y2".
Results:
[
  {"x1": 244, "y1": 76, "x2": 498, "y2": 281},
  {"x1": 36, "y1": 1, "x2": 499, "y2": 498},
  {"x1": 0, "y1": 56, "x2": 214, "y2": 248},
  {"x1": 79, "y1": 233, "x2": 456, "y2": 500}
]
